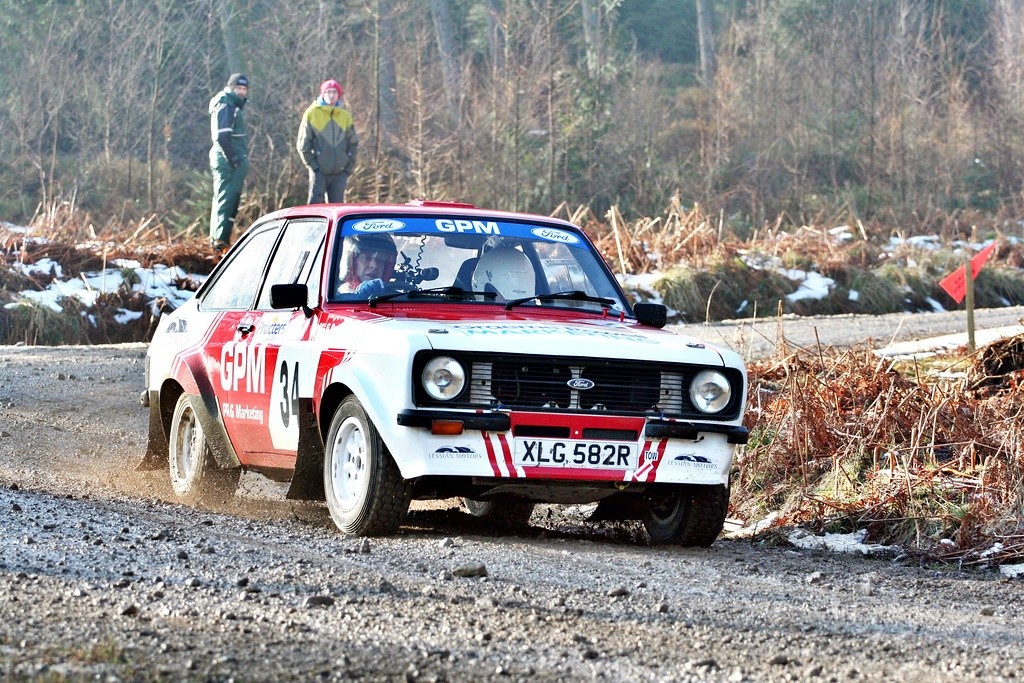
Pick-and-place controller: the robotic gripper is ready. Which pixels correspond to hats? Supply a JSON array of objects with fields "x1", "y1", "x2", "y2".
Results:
[
  {"x1": 320, "y1": 80, "x2": 342, "y2": 101},
  {"x1": 227, "y1": 73, "x2": 248, "y2": 86}
]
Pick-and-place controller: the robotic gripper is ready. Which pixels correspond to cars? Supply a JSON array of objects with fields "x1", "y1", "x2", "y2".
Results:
[{"x1": 134, "y1": 203, "x2": 751, "y2": 550}]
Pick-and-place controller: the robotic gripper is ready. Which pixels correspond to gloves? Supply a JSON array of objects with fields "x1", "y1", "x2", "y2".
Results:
[{"x1": 355, "y1": 279, "x2": 385, "y2": 297}]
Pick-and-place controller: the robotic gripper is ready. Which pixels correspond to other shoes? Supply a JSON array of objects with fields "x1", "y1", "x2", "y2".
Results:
[{"x1": 209, "y1": 239, "x2": 228, "y2": 250}]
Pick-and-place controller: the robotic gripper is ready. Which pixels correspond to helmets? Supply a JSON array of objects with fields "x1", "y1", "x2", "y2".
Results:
[
  {"x1": 471, "y1": 246, "x2": 536, "y2": 302},
  {"x1": 338, "y1": 233, "x2": 398, "y2": 289}
]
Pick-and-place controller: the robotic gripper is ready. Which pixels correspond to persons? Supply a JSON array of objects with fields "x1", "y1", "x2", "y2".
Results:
[
  {"x1": 297, "y1": 79, "x2": 359, "y2": 204},
  {"x1": 208, "y1": 73, "x2": 249, "y2": 251},
  {"x1": 336, "y1": 233, "x2": 399, "y2": 294},
  {"x1": 472, "y1": 247, "x2": 535, "y2": 303}
]
